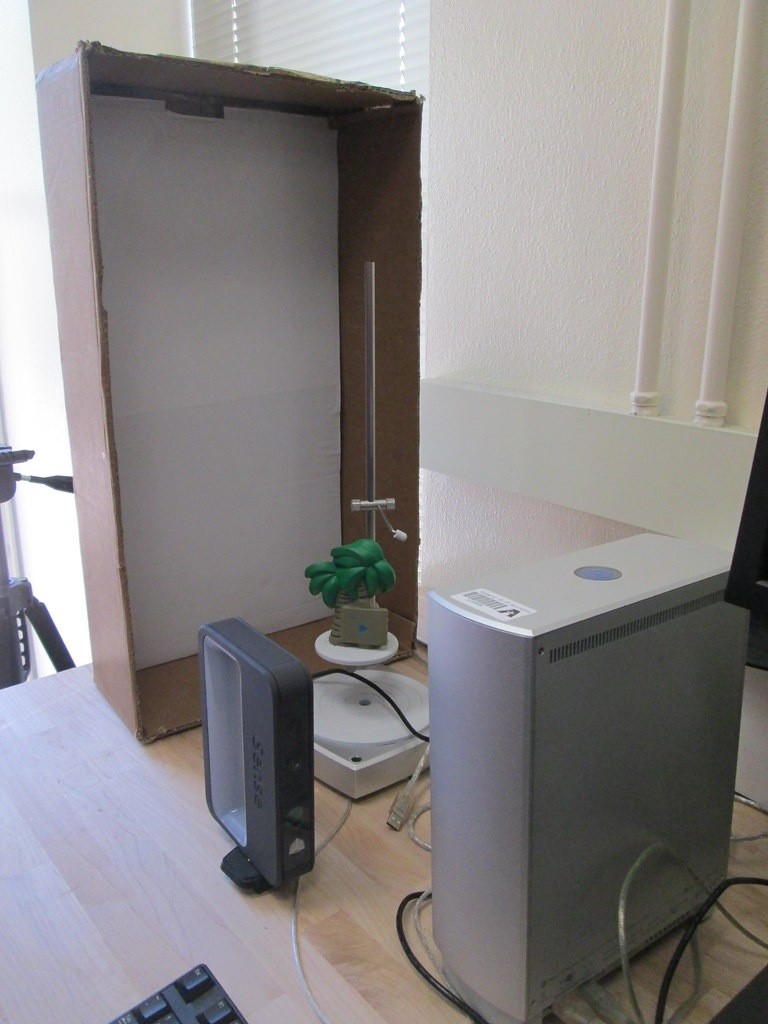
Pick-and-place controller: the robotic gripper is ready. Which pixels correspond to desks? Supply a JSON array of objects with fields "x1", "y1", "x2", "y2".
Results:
[{"x1": 0, "y1": 640, "x2": 768, "y2": 1024}]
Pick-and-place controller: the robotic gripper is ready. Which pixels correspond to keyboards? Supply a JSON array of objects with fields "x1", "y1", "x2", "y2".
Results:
[{"x1": 110, "y1": 964, "x2": 248, "y2": 1024}]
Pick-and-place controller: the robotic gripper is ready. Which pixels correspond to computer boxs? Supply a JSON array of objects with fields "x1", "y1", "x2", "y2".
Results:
[{"x1": 427, "y1": 532, "x2": 747, "y2": 1024}]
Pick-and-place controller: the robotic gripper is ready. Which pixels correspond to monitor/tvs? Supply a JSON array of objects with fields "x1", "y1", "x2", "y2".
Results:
[{"x1": 724, "y1": 389, "x2": 768, "y2": 613}]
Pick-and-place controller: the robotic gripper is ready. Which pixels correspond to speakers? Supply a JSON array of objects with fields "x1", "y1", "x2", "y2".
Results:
[{"x1": 196, "y1": 616, "x2": 316, "y2": 894}]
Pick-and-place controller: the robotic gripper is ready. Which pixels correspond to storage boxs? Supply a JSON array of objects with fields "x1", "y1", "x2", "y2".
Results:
[{"x1": 35, "y1": 41, "x2": 426, "y2": 748}]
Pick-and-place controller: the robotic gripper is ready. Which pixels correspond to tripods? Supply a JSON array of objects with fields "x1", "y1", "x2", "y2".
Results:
[{"x1": 0, "y1": 445, "x2": 76, "y2": 689}]
